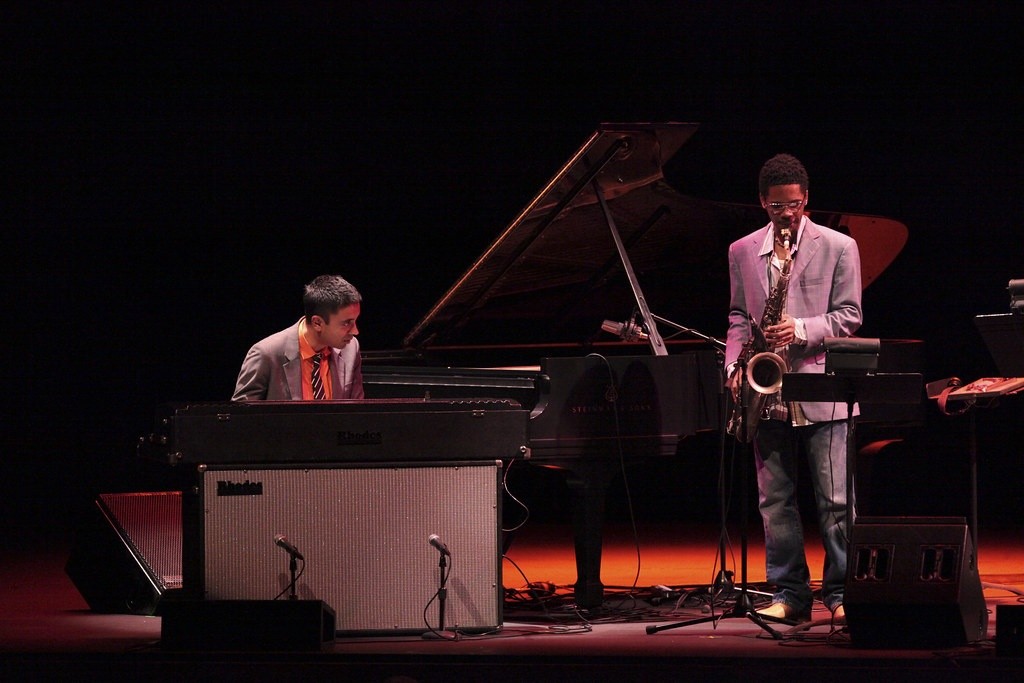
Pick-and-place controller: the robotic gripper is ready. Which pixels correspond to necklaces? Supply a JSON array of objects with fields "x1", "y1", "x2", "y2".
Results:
[{"x1": 775, "y1": 235, "x2": 785, "y2": 249}]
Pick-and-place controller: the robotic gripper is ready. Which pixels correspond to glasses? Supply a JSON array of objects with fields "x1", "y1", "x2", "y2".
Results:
[{"x1": 764, "y1": 194, "x2": 806, "y2": 214}]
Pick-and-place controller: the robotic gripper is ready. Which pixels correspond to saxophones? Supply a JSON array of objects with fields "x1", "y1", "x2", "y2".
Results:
[{"x1": 726, "y1": 221, "x2": 791, "y2": 440}]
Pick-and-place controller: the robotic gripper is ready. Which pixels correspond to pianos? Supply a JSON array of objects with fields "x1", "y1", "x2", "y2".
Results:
[
  {"x1": 161, "y1": 394, "x2": 533, "y2": 472},
  {"x1": 326, "y1": 113, "x2": 930, "y2": 617}
]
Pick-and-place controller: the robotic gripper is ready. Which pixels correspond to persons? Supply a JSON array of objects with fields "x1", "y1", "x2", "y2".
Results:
[
  {"x1": 231, "y1": 276, "x2": 365, "y2": 402},
  {"x1": 723, "y1": 153, "x2": 863, "y2": 625}
]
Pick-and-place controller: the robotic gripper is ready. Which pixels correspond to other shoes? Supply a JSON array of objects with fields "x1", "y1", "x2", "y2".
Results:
[
  {"x1": 755, "y1": 600, "x2": 812, "y2": 621},
  {"x1": 833, "y1": 605, "x2": 846, "y2": 622}
]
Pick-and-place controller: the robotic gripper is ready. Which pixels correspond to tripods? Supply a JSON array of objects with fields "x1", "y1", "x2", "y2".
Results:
[{"x1": 622, "y1": 303, "x2": 809, "y2": 640}]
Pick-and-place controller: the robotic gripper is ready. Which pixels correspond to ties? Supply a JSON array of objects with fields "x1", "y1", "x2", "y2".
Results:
[{"x1": 312, "y1": 353, "x2": 327, "y2": 401}]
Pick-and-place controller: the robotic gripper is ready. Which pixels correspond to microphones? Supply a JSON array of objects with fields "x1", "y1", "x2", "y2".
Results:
[
  {"x1": 428, "y1": 534, "x2": 452, "y2": 558},
  {"x1": 273, "y1": 534, "x2": 305, "y2": 559},
  {"x1": 601, "y1": 319, "x2": 650, "y2": 341},
  {"x1": 749, "y1": 313, "x2": 768, "y2": 352}
]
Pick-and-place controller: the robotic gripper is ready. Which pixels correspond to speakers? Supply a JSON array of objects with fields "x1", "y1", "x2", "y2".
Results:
[
  {"x1": 160, "y1": 598, "x2": 335, "y2": 650},
  {"x1": 64, "y1": 490, "x2": 184, "y2": 614},
  {"x1": 181, "y1": 458, "x2": 505, "y2": 637},
  {"x1": 842, "y1": 516, "x2": 989, "y2": 643}
]
